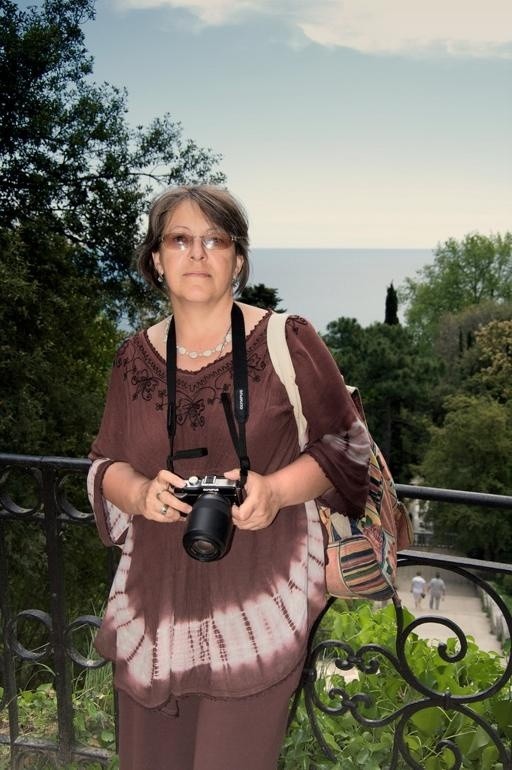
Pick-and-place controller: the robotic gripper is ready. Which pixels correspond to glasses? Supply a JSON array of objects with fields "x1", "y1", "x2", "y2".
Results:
[{"x1": 160, "y1": 232, "x2": 240, "y2": 251}]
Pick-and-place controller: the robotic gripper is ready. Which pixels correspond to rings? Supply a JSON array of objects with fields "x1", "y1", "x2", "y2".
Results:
[
  {"x1": 161, "y1": 505, "x2": 168, "y2": 515},
  {"x1": 157, "y1": 488, "x2": 169, "y2": 500}
]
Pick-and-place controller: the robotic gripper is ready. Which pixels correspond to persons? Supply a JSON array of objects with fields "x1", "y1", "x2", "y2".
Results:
[
  {"x1": 426, "y1": 572, "x2": 446, "y2": 611},
  {"x1": 87, "y1": 185, "x2": 369, "y2": 770},
  {"x1": 410, "y1": 572, "x2": 427, "y2": 609}
]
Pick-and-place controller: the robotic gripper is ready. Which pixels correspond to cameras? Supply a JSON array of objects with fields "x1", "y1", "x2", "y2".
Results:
[{"x1": 168, "y1": 475, "x2": 247, "y2": 565}]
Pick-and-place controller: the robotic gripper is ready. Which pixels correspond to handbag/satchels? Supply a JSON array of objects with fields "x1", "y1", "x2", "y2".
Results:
[{"x1": 316, "y1": 434, "x2": 413, "y2": 601}]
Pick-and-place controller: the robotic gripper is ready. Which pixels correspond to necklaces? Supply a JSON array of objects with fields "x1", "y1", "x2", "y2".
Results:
[{"x1": 164, "y1": 318, "x2": 232, "y2": 359}]
